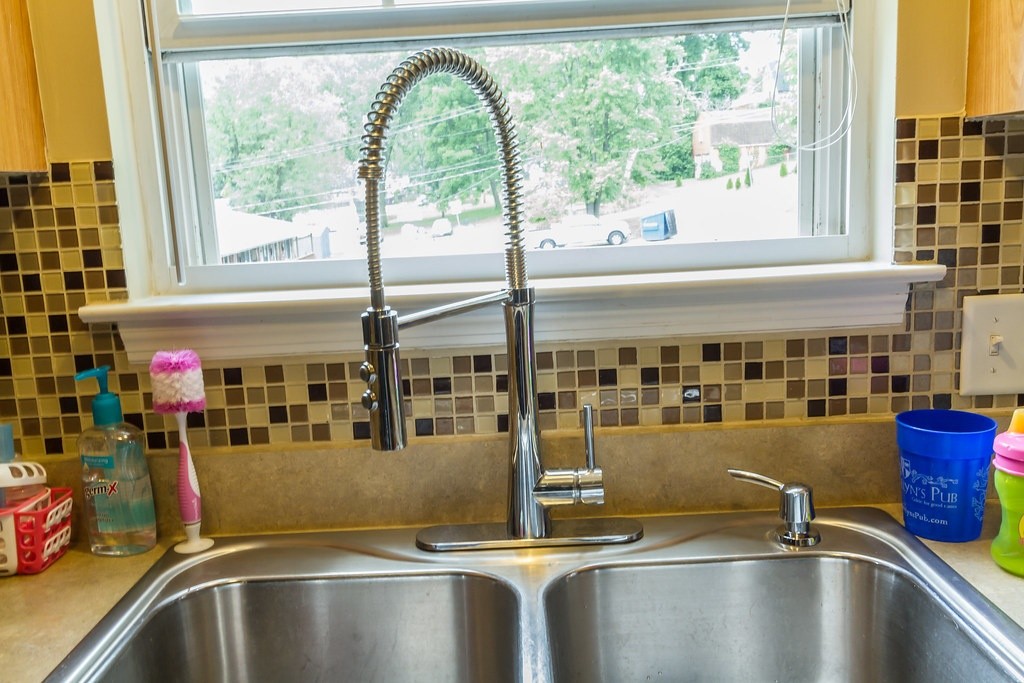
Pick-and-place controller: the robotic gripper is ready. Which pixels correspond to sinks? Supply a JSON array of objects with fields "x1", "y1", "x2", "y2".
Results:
[
  {"x1": 539, "y1": 549, "x2": 1023, "y2": 682},
  {"x1": 87, "y1": 569, "x2": 523, "y2": 683}
]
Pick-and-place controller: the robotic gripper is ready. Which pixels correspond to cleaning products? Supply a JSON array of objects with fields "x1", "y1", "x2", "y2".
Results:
[{"x1": 73, "y1": 363, "x2": 157, "y2": 557}]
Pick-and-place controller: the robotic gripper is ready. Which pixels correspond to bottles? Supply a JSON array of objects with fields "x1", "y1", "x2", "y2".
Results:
[{"x1": 990, "y1": 407, "x2": 1024, "y2": 579}]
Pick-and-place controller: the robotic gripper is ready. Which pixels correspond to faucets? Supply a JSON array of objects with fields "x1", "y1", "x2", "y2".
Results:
[{"x1": 356, "y1": 45, "x2": 605, "y2": 537}]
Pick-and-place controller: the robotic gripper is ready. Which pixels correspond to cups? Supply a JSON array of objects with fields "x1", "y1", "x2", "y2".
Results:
[{"x1": 895, "y1": 408, "x2": 998, "y2": 543}]
